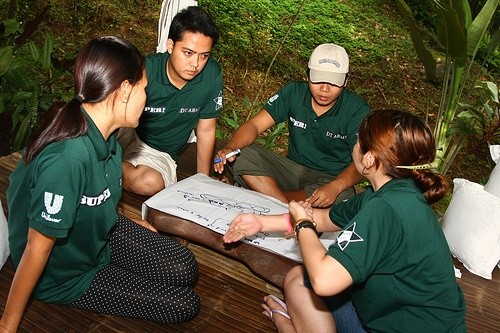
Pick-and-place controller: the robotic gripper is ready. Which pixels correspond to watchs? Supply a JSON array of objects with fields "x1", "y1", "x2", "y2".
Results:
[{"x1": 295, "y1": 221, "x2": 323, "y2": 241}]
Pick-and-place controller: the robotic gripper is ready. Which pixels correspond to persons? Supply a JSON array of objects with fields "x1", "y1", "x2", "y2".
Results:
[
  {"x1": 117, "y1": 5, "x2": 224, "y2": 197},
  {"x1": 214, "y1": 41, "x2": 374, "y2": 208},
  {"x1": 222, "y1": 109, "x2": 466, "y2": 332},
  {"x1": 0, "y1": 34, "x2": 201, "y2": 333}
]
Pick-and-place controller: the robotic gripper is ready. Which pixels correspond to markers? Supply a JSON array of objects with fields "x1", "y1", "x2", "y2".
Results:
[{"x1": 213, "y1": 148, "x2": 242, "y2": 164}]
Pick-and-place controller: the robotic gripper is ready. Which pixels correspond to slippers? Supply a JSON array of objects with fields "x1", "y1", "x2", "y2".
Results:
[{"x1": 268, "y1": 294, "x2": 292, "y2": 323}]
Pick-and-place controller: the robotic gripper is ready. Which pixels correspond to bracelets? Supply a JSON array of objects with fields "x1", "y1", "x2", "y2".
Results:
[
  {"x1": 282, "y1": 213, "x2": 294, "y2": 234},
  {"x1": 295, "y1": 218, "x2": 317, "y2": 228}
]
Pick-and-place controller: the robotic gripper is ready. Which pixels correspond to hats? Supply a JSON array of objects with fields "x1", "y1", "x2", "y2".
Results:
[{"x1": 307, "y1": 43, "x2": 350, "y2": 87}]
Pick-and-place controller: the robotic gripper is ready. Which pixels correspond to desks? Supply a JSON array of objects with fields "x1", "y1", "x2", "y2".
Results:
[{"x1": 141, "y1": 172, "x2": 342, "y2": 289}]
